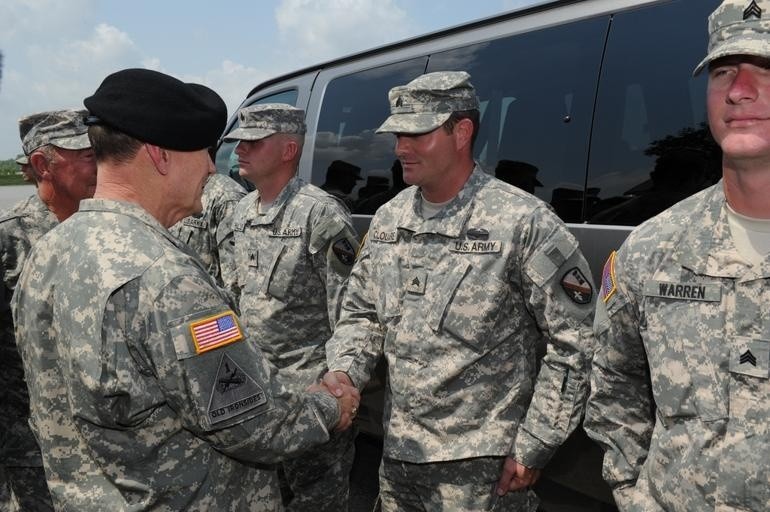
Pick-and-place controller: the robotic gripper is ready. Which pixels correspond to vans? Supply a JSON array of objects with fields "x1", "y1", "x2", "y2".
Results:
[{"x1": 206, "y1": 0, "x2": 723, "y2": 291}]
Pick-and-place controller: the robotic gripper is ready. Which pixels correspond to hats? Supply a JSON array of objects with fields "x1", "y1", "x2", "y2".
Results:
[
  {"x1": 497, "y1": 158, "x2": 542, "y2": 187},
  {"x1": 19, "y1": 108, "x2": 95, "y2": 153},
  {"x1": 330, "y1": 159, "x2": 364, "y2": 182},
  {"x1": 375, "y1": 71, "x2": 480, "y2": 134},
  {"x1": 223, "y1": 103, "x2": 307, "y2": 142},
  {"x1": 81, "y1": 68, "x2": 227, "y2": 152},
  {"x1": 692, "y1": 0, "x2": 770, "y2": 81}
]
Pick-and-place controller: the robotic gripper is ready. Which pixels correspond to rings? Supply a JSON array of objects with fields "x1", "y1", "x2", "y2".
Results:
[{"x1": 352, "y1": 407, "x2": 357, "y2": 415}]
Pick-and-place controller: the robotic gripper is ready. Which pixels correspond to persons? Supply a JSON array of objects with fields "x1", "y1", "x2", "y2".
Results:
[
  {"x1": 321, "y1": 68, "x2": 597, "y2": 511},
  {"x1": 15, "y1": 154, "x2": 35, "y2": 184},
  {"x1": 166, "y1": 172, "x2": 249, "y2": 319},
  {"x1": 1, "y1": 107, "x2": 96, "y2": 512},
  {"x1": 6, "y1": 68, "x2": 363, "y2": 512},
  {"x1": 580, "y1": 1, "x2": 770, "y2": 512},
  {"x1": 220, "y1": 102, "x2": 361, "y2": 510}
]
